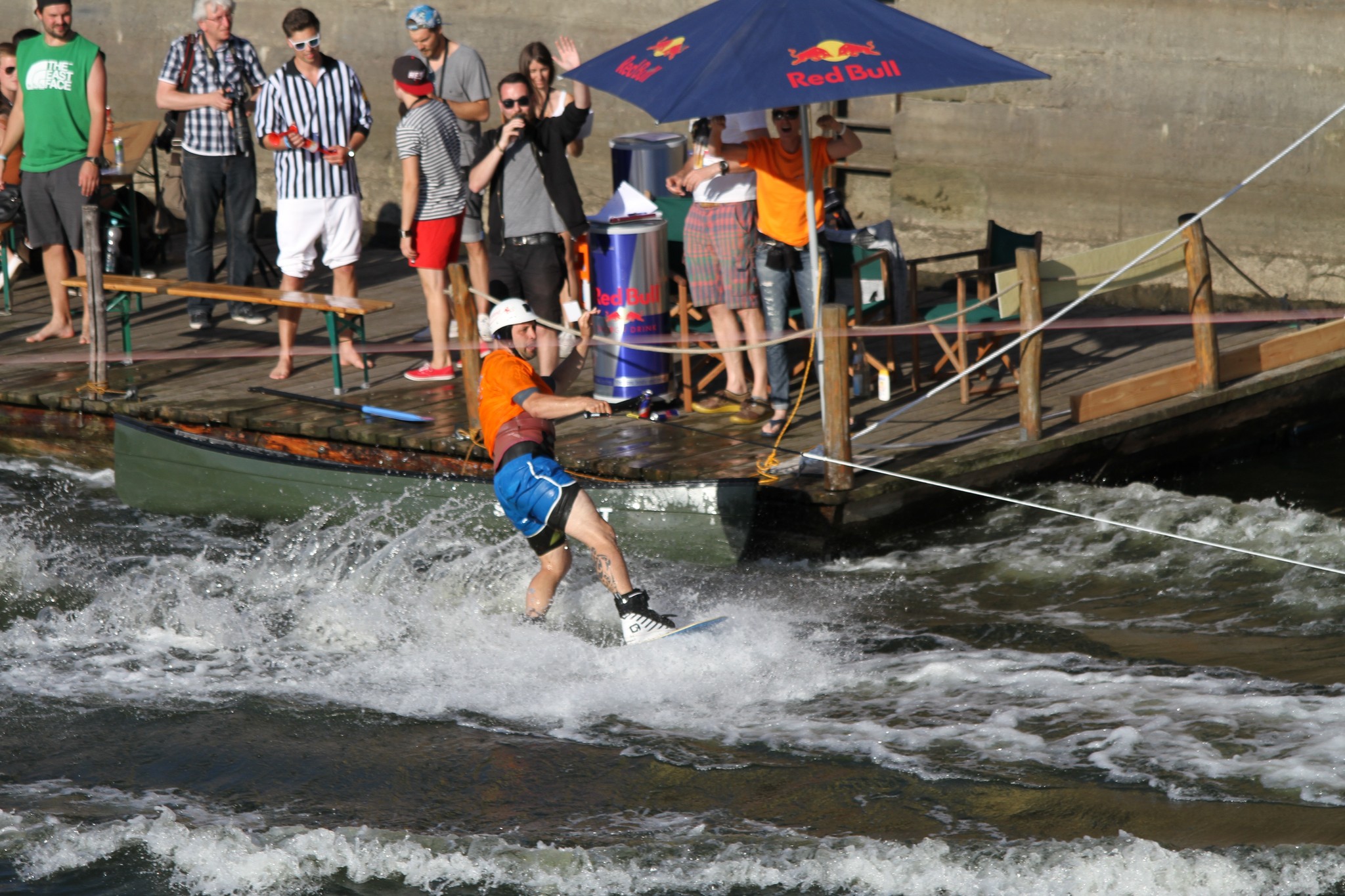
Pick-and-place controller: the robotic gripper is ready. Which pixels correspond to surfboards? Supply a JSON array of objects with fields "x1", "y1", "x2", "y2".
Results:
[{"x1": 601, "y1": 616, "x2": 730, "y2": 652}]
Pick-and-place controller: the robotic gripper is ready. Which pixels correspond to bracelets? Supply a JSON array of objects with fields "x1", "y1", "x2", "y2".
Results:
[
  {"x1": 283, "y1": 137, "x2": 292, "y2": 150},
  {"x1": 836, "y1": 122, "x2": 847, "y2": 139},
  {"x1": 0, "y1": 155, "x2": 7, "y2": 160},
  {"x1": 495, "y1": 143, "x2": 505, "y2": 157},
  {"x1": 396, "y1": 229, "x2": 410, "y2": 238}
]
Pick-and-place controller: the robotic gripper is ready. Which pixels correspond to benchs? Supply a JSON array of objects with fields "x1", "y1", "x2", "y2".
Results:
[{"x1": 62, "y1": 275, "x2": 396, "y2": 395}]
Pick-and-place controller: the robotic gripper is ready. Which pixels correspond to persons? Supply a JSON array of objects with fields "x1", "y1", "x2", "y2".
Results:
[
  {"x1": 519, "y1": 42, "x2": 584, "y2": 157},
  {"x1": 708, "y1": 102, "x2": 863, "y2": 437},
  {"x1": 155, "y1": 0, "x2": 268, "y2": 330},
  {"x1": 664, "y1": 112, "x2": 770, "y2": 426},
  {"x1": 254, "y1": 8, "x2": 375, "y2": 382},
  {"x1": 0, "y1": 0, "x2": 107, "y2": 345},
  {"x1": 467, "y1": 35, "x2": 591, "y2": 378},
  {"x1": 477, "y1": 299, "x2": 678, "y2": 646},
  {"x1": 404, "y1": 5, "x2": 495, "y2": 346},
  {"x1": 392, "y1": 56, "x2": 491, "y2": 381}
]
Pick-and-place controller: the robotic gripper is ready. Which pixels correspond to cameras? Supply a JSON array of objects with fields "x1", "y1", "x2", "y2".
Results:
[{"x1": 225, "y1": 93, "x2": 241, "y2": 105}]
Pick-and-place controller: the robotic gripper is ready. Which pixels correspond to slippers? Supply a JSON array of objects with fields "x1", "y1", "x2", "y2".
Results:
[{"x1": 760, "y1": 419, "x2": 791, "y2": 438}]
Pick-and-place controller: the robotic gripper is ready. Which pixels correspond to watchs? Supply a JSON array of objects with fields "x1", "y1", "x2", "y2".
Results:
[
  {"x1": 719, "y1": 161, "x2": 732, "y2": 176},
  {"x1": 348, "y1": 146, "x2": 356, "y2": 158},
  {"x1": 83, "y1": 156, "x2": 100, "y2": 166}
]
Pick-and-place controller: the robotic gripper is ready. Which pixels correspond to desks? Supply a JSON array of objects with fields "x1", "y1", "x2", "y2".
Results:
[{"x1": 98, "y1": 118, "x2": 166, "y2": 312}]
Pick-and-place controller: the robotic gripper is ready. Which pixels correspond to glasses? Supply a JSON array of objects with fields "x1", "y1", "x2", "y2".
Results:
[
  {"x1": 206, "y1": 12, "x2": 234, "y2": 22},
  {"x1": 288, "y1": 33, "x2": 320, "y2": 51},
  {"x1": 771, "y1": 109, "x2": 799, "y2": 121},
  {"x1": 0, "y1": 67, "x2": 16, "y2": 76},
  {"x1": 501, "y1": 93, "x2": 532, "y2": 109}
]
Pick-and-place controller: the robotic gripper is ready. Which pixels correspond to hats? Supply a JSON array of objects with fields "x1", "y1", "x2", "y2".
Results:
[
  {"x1": 404, "y1": 5, "x2": 441, "y2": 31},
  {"x1": 392, "y1": 56, "x2": 434, "y2": 96}
]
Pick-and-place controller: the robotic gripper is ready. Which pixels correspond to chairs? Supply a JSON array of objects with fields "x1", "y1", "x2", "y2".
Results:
[{"x1": 650, "y1": 194, "x2": 1043, "y2": 413}]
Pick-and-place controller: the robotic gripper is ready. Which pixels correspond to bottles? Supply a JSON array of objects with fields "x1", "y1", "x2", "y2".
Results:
[
  {"x1": 103, "y1": 219, "x2": 122, "y2": 273},
  {"x1": 877, "y1": 368, "x2": 890, "y2": 402}
]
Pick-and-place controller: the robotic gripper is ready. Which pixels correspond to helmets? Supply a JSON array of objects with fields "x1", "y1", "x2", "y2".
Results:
[{"x1": 489, "y1": 298, "x2": 539, "y2": 335}]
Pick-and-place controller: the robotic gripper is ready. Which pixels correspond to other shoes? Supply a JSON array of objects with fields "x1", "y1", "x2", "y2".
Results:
[
  {"x1": 189, "y1": 309, "x2": 211, "y2": 329},
  {"x1": 448, "y1": 321, "x2": 459, "y2": 339},
  {"x1": 0, "y1": 253, "x2": 24, "y2": 293},
  {"x1": 230, "y1": 308, "x2": 267, "y2": 325},
  {"x1": 692, "y1": 390, "x2": 752, "y2": 414},
  {"x1": 476, "y1": 313, "x2": 494, "y2": 343},
  {"x1": 729, "y1": 397, "x2": 774, "y2": 425}
]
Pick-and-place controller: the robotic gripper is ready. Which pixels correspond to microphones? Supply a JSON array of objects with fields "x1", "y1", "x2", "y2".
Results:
[{"x1": 510, "y1": 114, "x2": 524, "y2": 144}]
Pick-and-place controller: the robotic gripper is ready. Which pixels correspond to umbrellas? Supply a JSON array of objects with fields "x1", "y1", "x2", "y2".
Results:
[{"x1": 561, "y1": 0, "x2": 1054, "y2": 435}]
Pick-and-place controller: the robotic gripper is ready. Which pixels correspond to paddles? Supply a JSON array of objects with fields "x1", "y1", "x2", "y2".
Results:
[{"x1": 249, "y1": 387, "x2": 435, "y2": 422}]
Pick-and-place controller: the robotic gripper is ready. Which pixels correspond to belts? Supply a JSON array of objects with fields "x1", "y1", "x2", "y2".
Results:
[{"x1": 504, "y1": 234, "x2": 561, "y2": 246}]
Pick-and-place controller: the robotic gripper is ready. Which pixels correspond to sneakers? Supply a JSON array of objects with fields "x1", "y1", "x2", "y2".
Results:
[
  {"x1": 615, "y1": 590, "x2": 676, "y2": 646},
  {"x1": 404, "y1": 363, "x2": 456, "y2": 381},
  {"x1": 456, "y1": 342, "x2": 492, "y2": 368}
]
